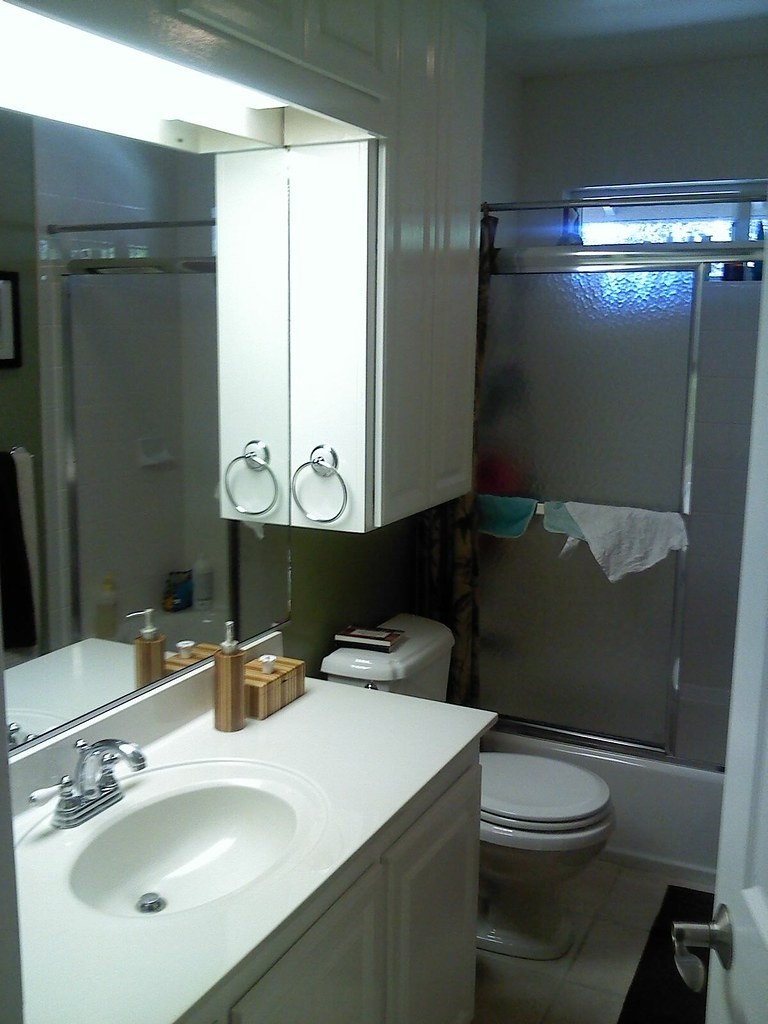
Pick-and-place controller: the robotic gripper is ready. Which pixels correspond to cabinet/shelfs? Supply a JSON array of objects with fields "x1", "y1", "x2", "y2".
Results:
[
  {"x1": 1, "y1": 0, "x2": 487, "y2": 536},
  {"x1": 181, "y1": 732, "x2": 486, "y2": 1024}
]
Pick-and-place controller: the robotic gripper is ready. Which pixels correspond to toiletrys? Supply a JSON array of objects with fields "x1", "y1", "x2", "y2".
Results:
[
  {"x1": 94, "y1": 572, "x2": 119, "y2": 640},
  {"x1": 126, "y1": 609, "x2": 167, "y2": 692},
  {"x1": 193, "y1": 552, "x2": 214, "y2": 611},
  {"x1": 213, "y1": 621, "x2": 247, "y2": 732}
]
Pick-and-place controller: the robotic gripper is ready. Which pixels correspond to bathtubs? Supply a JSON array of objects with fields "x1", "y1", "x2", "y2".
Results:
[
  {"x1": 89, "y1": 600, "x2": 284, "y2": 653},
  {"x1": 468, "y1": 640, "x2": 732, "y2": 895}
]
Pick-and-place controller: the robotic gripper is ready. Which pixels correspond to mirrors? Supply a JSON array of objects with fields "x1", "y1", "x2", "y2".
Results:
[{"x1": 0, "y1": 96, "x2": 296, "y2": 755}]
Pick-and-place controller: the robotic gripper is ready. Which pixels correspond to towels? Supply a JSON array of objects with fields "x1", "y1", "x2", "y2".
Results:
[
  {"x1": 542, "y1": 500, "x2": 689, "y2": 586},
  {"x1": 0, "y1": 449, "x2": 41, "y2": 666}
]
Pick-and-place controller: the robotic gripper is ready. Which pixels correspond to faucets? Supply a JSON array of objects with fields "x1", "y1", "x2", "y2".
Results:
[{"x1": 73, "y1": 738, "x2": 147, "y2": 802}]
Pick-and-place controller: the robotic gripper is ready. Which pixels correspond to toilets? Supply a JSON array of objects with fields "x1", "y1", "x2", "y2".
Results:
[{"x1": 318, "y1": 611, "x2": 615, "y2": 961}]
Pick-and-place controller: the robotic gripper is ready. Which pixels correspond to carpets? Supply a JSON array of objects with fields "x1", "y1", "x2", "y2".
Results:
[{"x1": 618, "y1": 884, "x2": 716, "y2": 1024}]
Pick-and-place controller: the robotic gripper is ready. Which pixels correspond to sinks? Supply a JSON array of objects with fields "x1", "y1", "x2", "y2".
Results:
[
  {"x1": 4, "y1": 707, "x2": 69, "y2": 750},
  {"x1": 14, "y1": 759, "x2": 330, "y2": 947}
]
